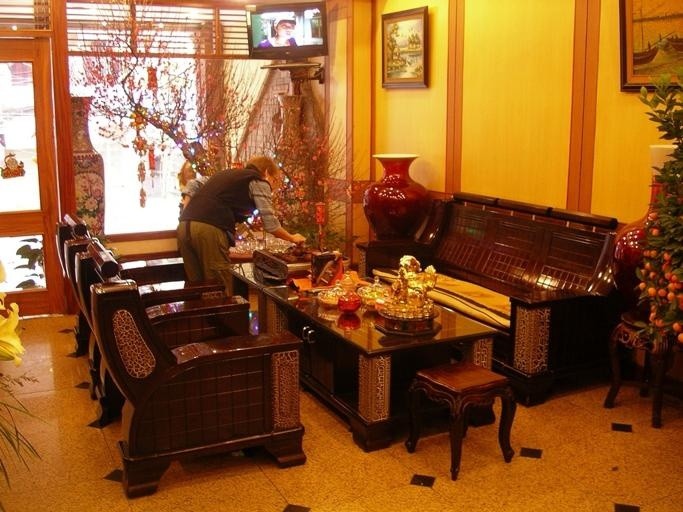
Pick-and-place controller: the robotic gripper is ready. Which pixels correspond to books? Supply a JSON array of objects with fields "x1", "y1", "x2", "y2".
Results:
[{"x1": 286, "y1": 271, "x2": 367, "y2": 293}]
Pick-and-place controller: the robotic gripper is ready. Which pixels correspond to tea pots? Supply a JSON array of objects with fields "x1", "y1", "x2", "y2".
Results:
[{"x1": 293, "y1": 246, "x2": 304, "y2": 257}]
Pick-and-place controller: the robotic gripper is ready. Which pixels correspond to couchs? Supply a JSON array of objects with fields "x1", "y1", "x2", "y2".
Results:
[{"x1": 355, "y1": 190, "x2": 619, "y2": 408}]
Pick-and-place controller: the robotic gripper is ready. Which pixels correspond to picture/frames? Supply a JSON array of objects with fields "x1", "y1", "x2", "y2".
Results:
[
  {"x1": 380, "y1": 6, "x2": 429, "y2": 90},
  {"x1": 619, "y1": 0, "x2": 683, "y2": 92}
]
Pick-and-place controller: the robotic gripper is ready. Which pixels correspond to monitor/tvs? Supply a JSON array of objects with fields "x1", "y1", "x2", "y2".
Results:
[{"x1": 245, "y1": 0, "x2": 329, "y2": 70}]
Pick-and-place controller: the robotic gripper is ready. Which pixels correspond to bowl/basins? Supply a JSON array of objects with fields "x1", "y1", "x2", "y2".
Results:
[
  {"x1": 338, "y1": 294, "x2": 361, "y2": 313},
  {"x1": 337, "y1": 312, "x2": 361, "y2": 331}
]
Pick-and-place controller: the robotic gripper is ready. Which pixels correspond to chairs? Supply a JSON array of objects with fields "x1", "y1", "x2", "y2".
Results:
[
  {"x1": 73, "y1": 237, "x2": 307, "y2": 499},
  {"x1": 55, "y1": 212, "x2": 226, "y2": 400}
]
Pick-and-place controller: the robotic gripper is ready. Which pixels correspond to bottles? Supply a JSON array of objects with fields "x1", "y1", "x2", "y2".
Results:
[{"x1": 316, "y1": 255, "x2": 341, "y2": 286}]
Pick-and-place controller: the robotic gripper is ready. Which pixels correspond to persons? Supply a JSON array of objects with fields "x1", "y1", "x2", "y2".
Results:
[
  {"x1": 177, "y1": 155, "x2": 306, "y2": 299},
  {"x1": 257, "y1": 12, "x2": 296, "y2": 47}
]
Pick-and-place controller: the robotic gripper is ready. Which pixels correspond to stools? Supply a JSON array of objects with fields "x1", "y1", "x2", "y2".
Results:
[
  {"x1": 405, "y1": 361, "x2": 517, "y2": 482},
  {"x1": 603, "y1": 312, "x2": 677, "y2": 428}
]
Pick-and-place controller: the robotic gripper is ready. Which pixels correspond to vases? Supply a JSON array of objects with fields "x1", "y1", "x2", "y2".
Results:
[
  {"x1": 363, "y1": 154, "x2": 429, "y2": 242},
  {"x1": 277, "y1": 94, "x2": 306, "y2": 138},
  {"x1": 70, "y1": 96, "x2": 104, "y2": 235}
]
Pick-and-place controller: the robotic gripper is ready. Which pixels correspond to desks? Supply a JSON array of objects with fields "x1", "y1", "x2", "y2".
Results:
[
  {"x1": 263, "y1": 288, "x2": 500, "y2": 451},
  {"x1": 355, "y1": 240, "x2": 368, "y2": 277},
  {"x1": 228, "y1": 262, "x2": 313, "y2": 335}
]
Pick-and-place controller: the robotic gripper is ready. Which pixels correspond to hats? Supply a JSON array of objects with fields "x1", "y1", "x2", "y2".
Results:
[{"x1": 274, "y1": 18, "x2": 296, "y2": 29}]
